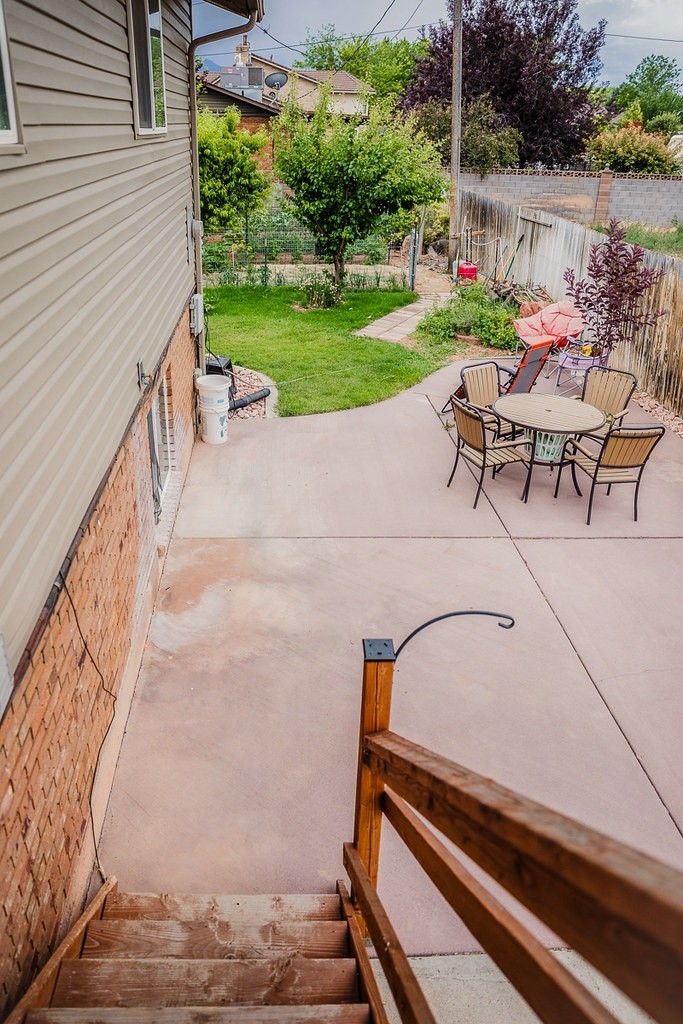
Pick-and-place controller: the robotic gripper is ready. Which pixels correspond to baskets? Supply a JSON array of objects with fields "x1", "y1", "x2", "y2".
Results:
[{"x1": 523, "y1": 427, "x2": 570, "y2": 461}]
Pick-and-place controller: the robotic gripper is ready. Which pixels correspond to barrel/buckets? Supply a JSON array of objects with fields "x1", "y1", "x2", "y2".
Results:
[
  {"x1": 196, "y1": 374, "x2": 231, "y2": 445},
  {"x1": 458, "y1": 262, "x2": 478, "y2": 281}
]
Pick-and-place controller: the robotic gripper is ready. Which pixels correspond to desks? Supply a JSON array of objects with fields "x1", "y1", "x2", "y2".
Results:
[{"x1": 492, "y1": 393, "x2": 606, "y2": 505}]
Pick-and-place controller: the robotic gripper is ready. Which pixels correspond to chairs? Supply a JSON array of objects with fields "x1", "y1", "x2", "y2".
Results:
[
  {"x1": 554, "y1": 335, "x2": 614, "y2": 387},
  {"x1": 460, "y1": 361, "x2": 525, "y2": 464},
  {"x1": 572, "y1": 366, "x2": 638, "y2": 457},
  {"x1": 513, "y1": 299, "x2": 586, "y2": 379},
  {"x1": 554, "y1": 425, "x2": 666, "y2": 526},
  {"x1": 444, "y1": 393, "x2": 533, "y2": 510},
  {"x1": 442, "y1": 338, "x2": 554, "y2": 416}
]
